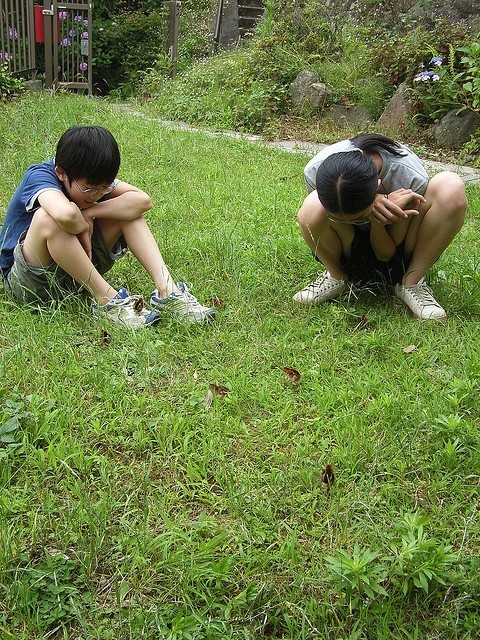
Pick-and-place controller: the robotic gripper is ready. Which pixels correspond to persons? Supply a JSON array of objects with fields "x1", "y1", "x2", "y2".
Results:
[
  {"x1": 0, "y1": 126, "x2": 216, "y2": 334},
  {"x1": 291, "y1": 133, "x2": 469, "y2": 323}
]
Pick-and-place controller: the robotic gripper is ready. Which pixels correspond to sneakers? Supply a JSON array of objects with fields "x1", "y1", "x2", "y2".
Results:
[
  {"x1": 92, "y1": 286, "x2": 161, "y2": 334},
  {"x1": 394, "y1": 277, "x2": 447, "y2": 324},
  {"x1": 149, "y1": 282, "x2": 216, "y2": 327},
  {"x1": 292, "y1": 269, "x2": 349, "y2": 309}
]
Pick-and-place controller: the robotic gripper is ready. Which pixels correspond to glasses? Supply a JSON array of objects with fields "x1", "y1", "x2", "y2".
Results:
[
  {"x1": 64, "y1": 170, "x2": 115, "y2": 198},
  {"x1": 326, "y1": 211, "x2": 373, "y2": 226}
]
